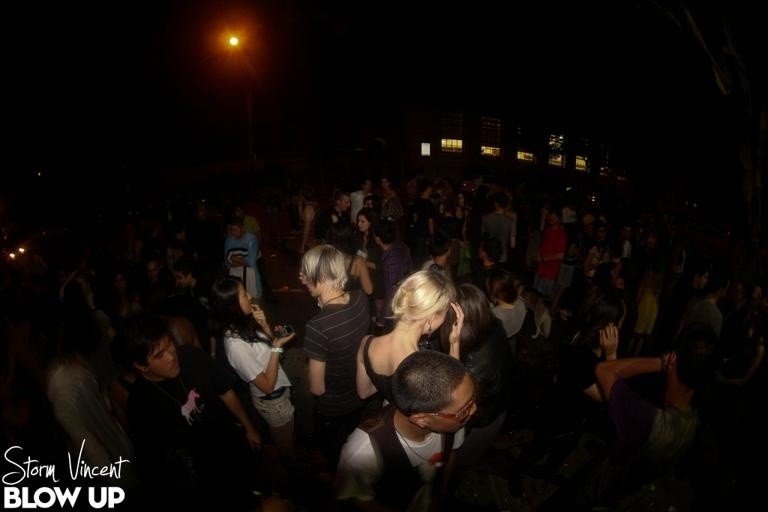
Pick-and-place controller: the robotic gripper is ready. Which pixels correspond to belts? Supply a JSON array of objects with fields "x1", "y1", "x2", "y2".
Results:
[{"x1": 260, "y1": 387, "x2": 287, "y2": 401}]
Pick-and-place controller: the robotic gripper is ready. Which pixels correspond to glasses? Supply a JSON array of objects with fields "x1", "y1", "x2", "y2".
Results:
[{"x1": 412, "y1": 374, "x2": 479, "y2": 422}]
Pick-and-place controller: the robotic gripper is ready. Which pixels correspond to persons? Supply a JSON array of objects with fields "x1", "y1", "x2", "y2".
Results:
[{"x1": 43, "y1": 171, "x2": 768, "y2": 512}]
